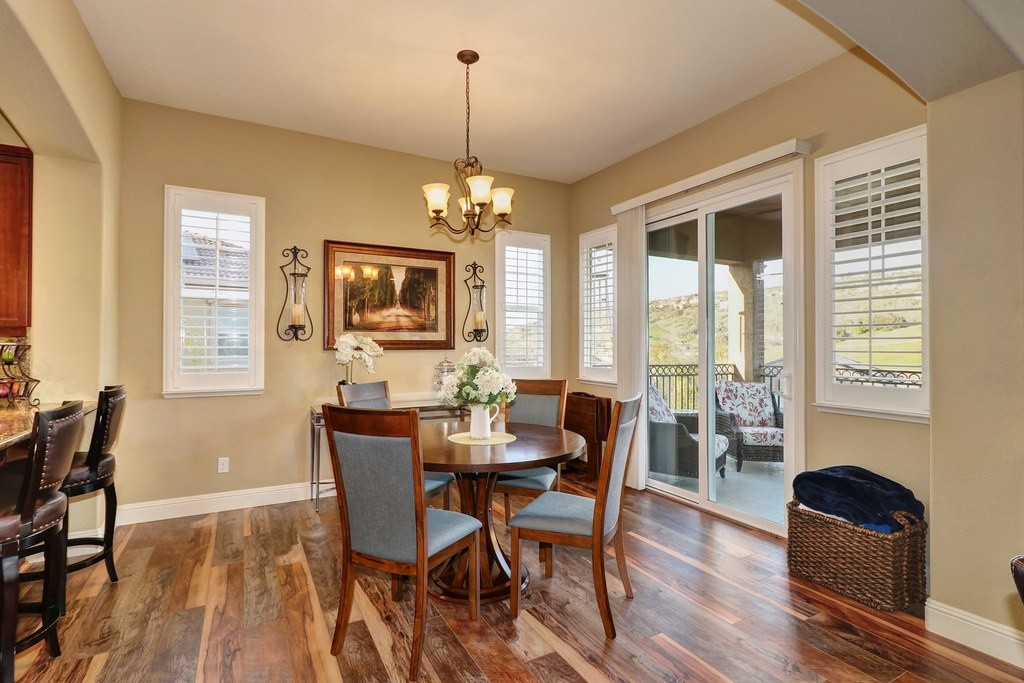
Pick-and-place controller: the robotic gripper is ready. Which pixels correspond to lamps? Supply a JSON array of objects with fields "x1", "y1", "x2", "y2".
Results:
[
  {"x1": 422, "y1": 50, "x2": 514, "y2": 236},
  {"x1": 462, "y1": 260, "x2": 490, "y2": 343},
  {"x1": 276, "y1": 245, "x2": 313, "y2": 340}
]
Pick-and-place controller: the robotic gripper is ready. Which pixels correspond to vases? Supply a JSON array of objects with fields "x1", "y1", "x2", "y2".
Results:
[{"x1": 337, "y1": 384, "x2": 345, "y2": 407}]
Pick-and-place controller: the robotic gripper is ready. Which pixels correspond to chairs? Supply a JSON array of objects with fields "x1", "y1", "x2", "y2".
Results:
[
  {"x1": 18, "y1": 385, "x2": 128, "y2": 617},
  {"x1": 0, "y1": 401, "x2": 84, "y2": 683},
  {"x1": 322, "y1": 381, "x2": 482, "y2": 680},
  {"x1": 648, "y1": 384, "x2": 729, "y2": 479},
  {"x1": 474, "y1": 378, "x2": 569, "y2": 562},
  {"x1": 716, "y1": 380, "x2": 784, "y2": 471},
  {"x1": 506, "y1": 393, "x2": 643, "y2": 638}
]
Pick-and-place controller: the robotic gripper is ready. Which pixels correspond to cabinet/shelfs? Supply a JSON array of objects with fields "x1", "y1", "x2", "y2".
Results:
[{"x1": 0, "y1": 143, "x2": 34, "y2": 338}]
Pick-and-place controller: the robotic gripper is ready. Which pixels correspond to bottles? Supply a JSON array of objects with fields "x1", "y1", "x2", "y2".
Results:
[{"x1": 435, "y1": 358, "x2": 456, "y2": 399}]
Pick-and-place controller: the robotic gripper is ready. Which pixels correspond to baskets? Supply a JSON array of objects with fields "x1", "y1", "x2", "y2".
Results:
[{"x1": 786, "y1": 491, "x2": 926, "y2": 613}]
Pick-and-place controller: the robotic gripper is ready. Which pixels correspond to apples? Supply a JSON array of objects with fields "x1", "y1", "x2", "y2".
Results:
[
  {"x1": 0, "y1": 382, "x2": 20, "y2": 398},
  {"x1": 2, "y1": 352, "x2": 14, "y2": 364}
]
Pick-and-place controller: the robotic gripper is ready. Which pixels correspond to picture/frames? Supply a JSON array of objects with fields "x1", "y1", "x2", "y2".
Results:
[
  {"x1": 468, "y1": 404, "x2": 500, "y2": 439},
  {"x1": 322, "y1": 239, "x2": 456, "y2": 351}
]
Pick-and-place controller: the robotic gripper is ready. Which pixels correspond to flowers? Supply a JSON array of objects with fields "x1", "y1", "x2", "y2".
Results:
[
  {"x1": 334, "y1": 334, "x2": 383, "y2": 384},
  {"x1": 439, "y1": 346, "x2": 517, "y2": 411}
]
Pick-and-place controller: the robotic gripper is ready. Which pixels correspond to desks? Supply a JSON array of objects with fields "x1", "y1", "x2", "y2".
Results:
[
  {"x1": 310, "y1": 400, "x2": 471, "y2": 511},
  {"x1": 420, "y1": 422, "x2": 586, "y2": 604},
  {"x1": 0, "y1": 402, "x2": 99, "y2": 467}
]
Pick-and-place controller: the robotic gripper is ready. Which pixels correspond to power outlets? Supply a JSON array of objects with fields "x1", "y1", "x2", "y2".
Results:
[{"x1": 218, "y1": 457, "x2": 230, "y2": 474}]
